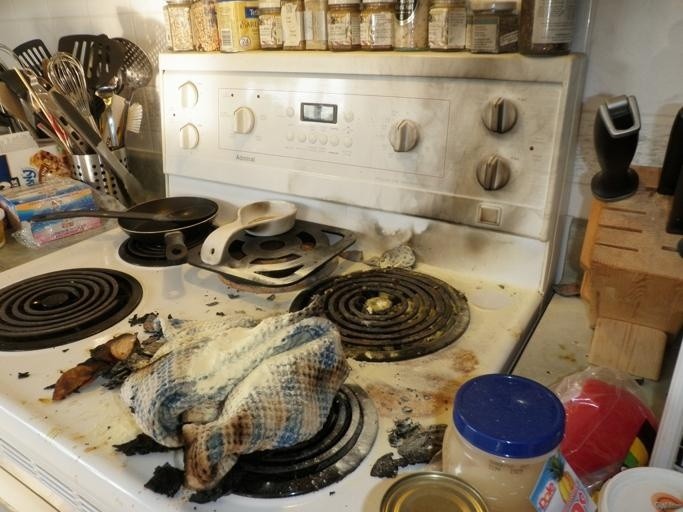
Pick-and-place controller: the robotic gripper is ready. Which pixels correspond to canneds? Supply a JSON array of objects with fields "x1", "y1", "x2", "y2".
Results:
[{"x1": 379, "y1": 474, "x2": 487, "y2": 512}]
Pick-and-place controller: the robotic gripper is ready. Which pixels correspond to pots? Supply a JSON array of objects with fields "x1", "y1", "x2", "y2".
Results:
[{"x1": 112, "y1": 193, "x2": 220, "y2": 265}]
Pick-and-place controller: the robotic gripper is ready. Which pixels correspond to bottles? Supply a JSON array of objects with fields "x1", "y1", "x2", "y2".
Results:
[{"x1": 438, "y1": 374, "x2": 568, "y2": 512}]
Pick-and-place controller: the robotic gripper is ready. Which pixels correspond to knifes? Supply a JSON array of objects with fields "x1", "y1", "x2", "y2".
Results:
[{"x1": 51, "y1": 86, "x2": 147, "y2": 204}]
